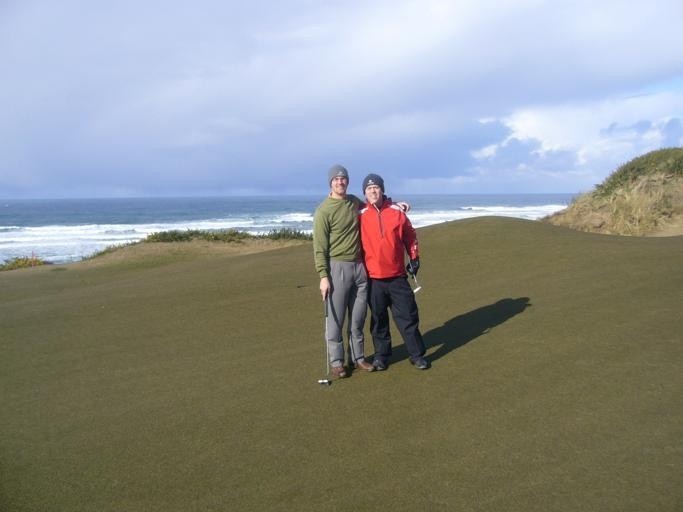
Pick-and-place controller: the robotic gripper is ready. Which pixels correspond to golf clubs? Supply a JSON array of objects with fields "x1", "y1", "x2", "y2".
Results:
[
  {"x1": 317, "y1": 289, "x2": 332, "y2": 386},
  {"x1": 405, "y1": 247, "x2": 422, "y2": 294}
]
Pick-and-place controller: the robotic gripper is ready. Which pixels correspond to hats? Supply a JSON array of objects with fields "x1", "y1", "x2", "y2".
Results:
[
  {"x1": 327, "y1": 164, "x2": 349, "y2": 188},
  {"x1": 362, "y1": 174, "x2": 384, "y2": 194}
]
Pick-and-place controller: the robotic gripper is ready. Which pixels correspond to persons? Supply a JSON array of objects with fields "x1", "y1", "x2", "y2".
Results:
[
  {"x1": 355, "y1": 172, "x2": 432, "y2": 372},
  {"x1": 310, "y1": 164, "x2": 413, "y2": 379}
]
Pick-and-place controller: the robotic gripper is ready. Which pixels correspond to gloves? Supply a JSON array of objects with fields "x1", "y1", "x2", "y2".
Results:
[{"x1": 406, "y1": 259, "x2": 419, "y2": 279}]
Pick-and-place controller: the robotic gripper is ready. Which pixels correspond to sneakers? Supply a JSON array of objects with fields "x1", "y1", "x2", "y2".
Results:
[
  {"x1": 372, "y1": 357, "x2": 389, "y2": 370},
  {"x1": 349, "y1": 359, "x2": 376, "y2": 372},
  {"x1": 332, "y1": 366, "x2": 348, "y2": 378},
  {"x1": 410, "y1": 356, "x2": 427, "y2": 369}
]
